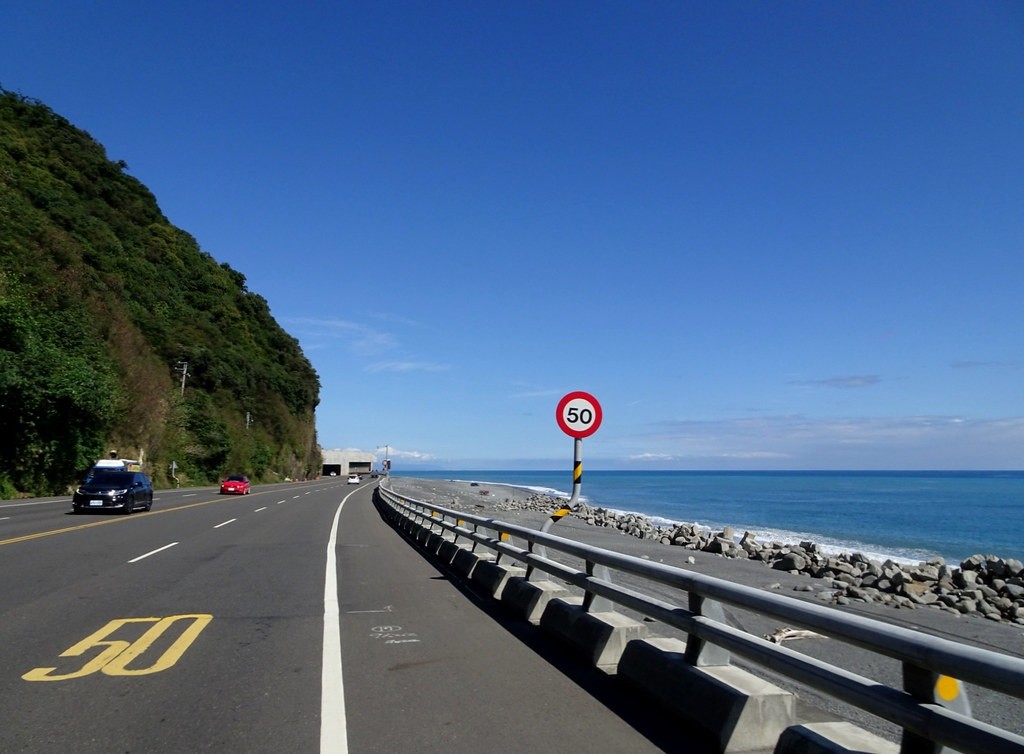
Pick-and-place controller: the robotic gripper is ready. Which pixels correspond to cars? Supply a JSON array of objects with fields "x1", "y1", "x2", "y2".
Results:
[
  {"x1": 72, "y1": 460, "x2": 153, "y2": 515},
  {"x1": 330, "y1": 471, "x2": 337, "y2": 477},
  {"x1": 220, "y1": 475, "x2": 251, "y2": 495},
  {"x1": 347, "y1": 473, "x2": 360, "y2": 485},
  {"x1": 358, "y1": 474, "x2": 363, "y2": 480}
]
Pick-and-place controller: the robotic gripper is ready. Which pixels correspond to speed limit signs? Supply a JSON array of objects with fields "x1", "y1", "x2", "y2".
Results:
[{"x1": 556, "y1": 390, "x2": 603, "y2": 439}]
